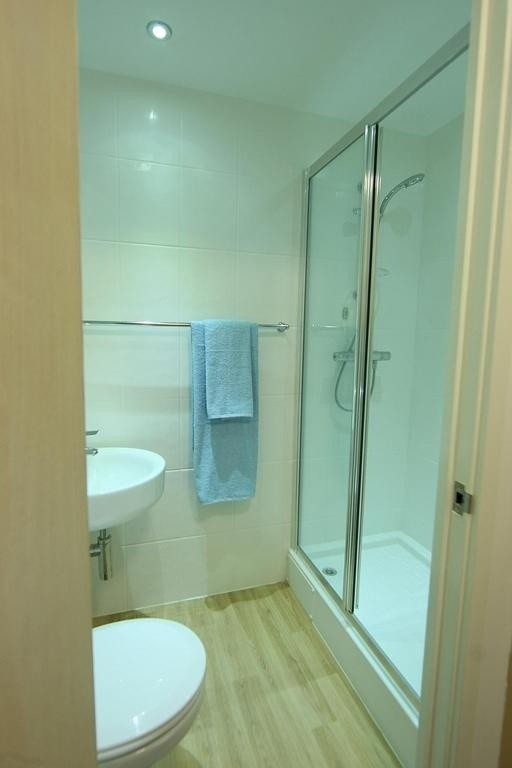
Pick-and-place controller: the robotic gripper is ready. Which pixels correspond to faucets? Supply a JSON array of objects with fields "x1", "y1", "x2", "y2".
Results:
[{"x1": 86, "y1": 430, "x2": 100, "y2": 455}]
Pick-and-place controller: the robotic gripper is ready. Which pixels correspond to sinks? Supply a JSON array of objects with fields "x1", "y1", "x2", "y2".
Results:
[{"x1": 84, "y1": 446, "x2": 166, "y2": 531}]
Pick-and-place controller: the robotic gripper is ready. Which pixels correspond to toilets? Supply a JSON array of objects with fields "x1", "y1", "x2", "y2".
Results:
[{"x1": 91, "y1": 618, "x2": 208, "y2": 765}]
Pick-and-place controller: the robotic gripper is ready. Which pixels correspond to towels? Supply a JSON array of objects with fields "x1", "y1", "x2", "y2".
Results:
[{"x1": 192, "y1": 319, "x2": 258, "y2": 504}]
